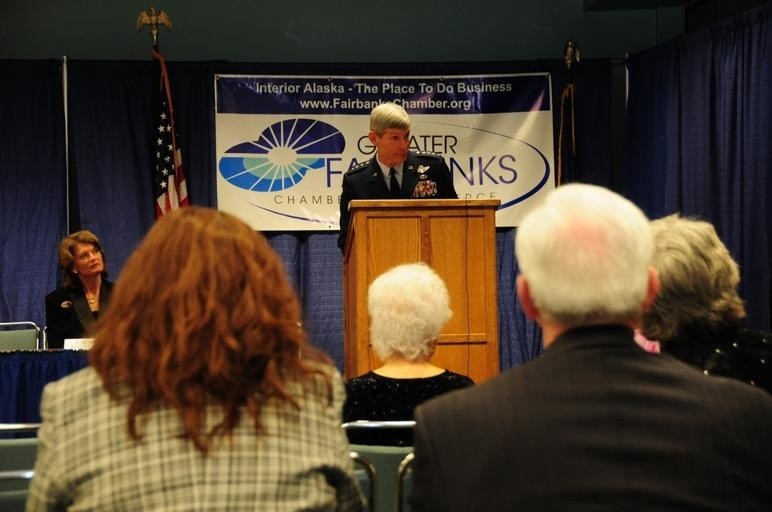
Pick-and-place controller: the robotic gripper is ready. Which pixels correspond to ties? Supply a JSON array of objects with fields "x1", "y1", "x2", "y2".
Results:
[{"x1": 390, "y1": 168, "x2": 401, "y2": 199}]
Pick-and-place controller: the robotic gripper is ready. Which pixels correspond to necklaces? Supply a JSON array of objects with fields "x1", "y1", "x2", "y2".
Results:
[{"x1": 86, "y1": 297, "x2": 99, "y2": 303}]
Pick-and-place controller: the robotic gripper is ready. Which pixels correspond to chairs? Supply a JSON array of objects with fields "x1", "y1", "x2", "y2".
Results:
[
  {"x1": 0, "y1": 322, "x2": 39, "y2": 352},
  {"x1": 0, "y1": 470, "x2": 34, "y2": 512},
  {"x1": 42, "y1": 326, "x2": 49, "y2": 349},
  {"x1": 395, "y1": 451, "x2": 415, "y2": 512},
  {"x1": 342, "y1": 418, "x2": 418, "y2": 512},
  {"x1": 1, "y1": 423, "x2": 54, "y2": 470},
  {"x1": 349, "y1": 452, "x2": 378, "y2": 512}
]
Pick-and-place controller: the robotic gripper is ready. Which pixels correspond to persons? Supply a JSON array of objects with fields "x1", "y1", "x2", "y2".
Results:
[
  {"x1": 342, "y1": 262, "x2": 476, "y2": 447},
  {"x1": 26, "y1": 205, "x2": 368, "y2": 512},
  {"x1": 45, "y1": 230, "x2": 114, "y2": 349},
  {"x1": 641, "y1": 213, "x2": 771, "y2": 396},
  {"x1": 336, "y1": 102, "x2": 459, "y2": 253},
  {"x1": 408, "y1": 182, "x2": 770, "y2": 512}
]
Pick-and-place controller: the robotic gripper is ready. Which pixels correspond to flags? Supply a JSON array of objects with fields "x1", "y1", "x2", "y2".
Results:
[{"x1": 153, "y1": 90, "x2": 190, "y2": 220}]
